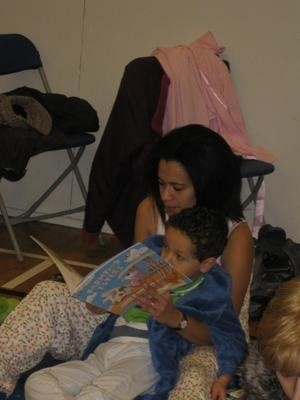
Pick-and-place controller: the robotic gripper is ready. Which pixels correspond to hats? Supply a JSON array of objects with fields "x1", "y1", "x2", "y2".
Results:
[{"x1": 1, "y1": 95, "x2": 53, "y2": 135}]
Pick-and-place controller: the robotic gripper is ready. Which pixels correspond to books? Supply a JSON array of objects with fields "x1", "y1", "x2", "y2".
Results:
[{"x1": 27, "y1": 235, "x2": 192, "y2": 316}]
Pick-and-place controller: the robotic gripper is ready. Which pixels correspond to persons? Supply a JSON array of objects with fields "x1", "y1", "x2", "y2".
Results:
[
  {"x1": 258, "y1": 276, "x2": 300, "y2": 400},
  {"x1": 24, "y1": 208, "x2": 250, "y2": 400},
  {"x1": 1, "y1": 125, "x2": 254, "y2": 400}
]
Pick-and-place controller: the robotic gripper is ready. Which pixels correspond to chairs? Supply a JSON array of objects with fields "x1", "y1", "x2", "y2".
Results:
[
  {"x1": 126, "y1": 52, "x2": 275, "y2": 225},
  {"x1": 0, "y1": 33, "x2": 106, "y2": 262}
]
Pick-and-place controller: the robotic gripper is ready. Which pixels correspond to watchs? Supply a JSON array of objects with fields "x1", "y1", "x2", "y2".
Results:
[{"x1": 173, "y1": 312, "x2": 188, "y2": 332}]
